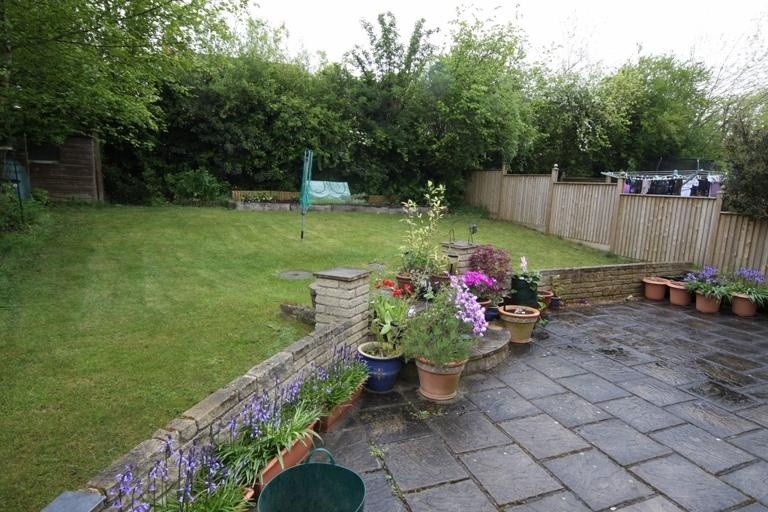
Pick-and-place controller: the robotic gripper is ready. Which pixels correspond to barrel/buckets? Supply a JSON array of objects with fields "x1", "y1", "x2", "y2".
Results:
[
  {"x1": 511, "y1": 275, "x2": 539, "y2": 308},
  {"x1": 259, "y1": 450, "x2": 366, "y2": 512}
]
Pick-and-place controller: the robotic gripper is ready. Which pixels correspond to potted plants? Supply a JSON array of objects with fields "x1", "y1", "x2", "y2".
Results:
[
  {"x1": 551, "y1": 287, "x2": 564, "y2": 307},
  {"x1": 396, "y1": 180, "x2": 447, "y2": 297}
]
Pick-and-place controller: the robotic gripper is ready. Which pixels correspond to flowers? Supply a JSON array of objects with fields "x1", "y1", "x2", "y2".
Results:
[
  {"x1": 728, "y1": 267, "x2": 767, "y2": 304},
  {"x1": 105, "y1": 255, "x2": 541, "y2": 512},
  {"x1": 684, "y1": 264, "x2": 730, "y2": 300}
]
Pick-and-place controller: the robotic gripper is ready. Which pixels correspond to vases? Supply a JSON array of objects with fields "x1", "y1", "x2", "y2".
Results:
[
  {"x1": 729, "y1": 292, "x2": 756, "y2": 317},
  {"x1": 695, "y1": 290, "x2": 721, "y2": 314},
  {"x1": 666, "y1": 280, "x2": 690, "y2": 306},
  {"x1": 643, "y1": 277, "x2": 669, "y2": 300},
  {"x1": 537, "y1": 291, "x2": 554, "y2": 314}
]
